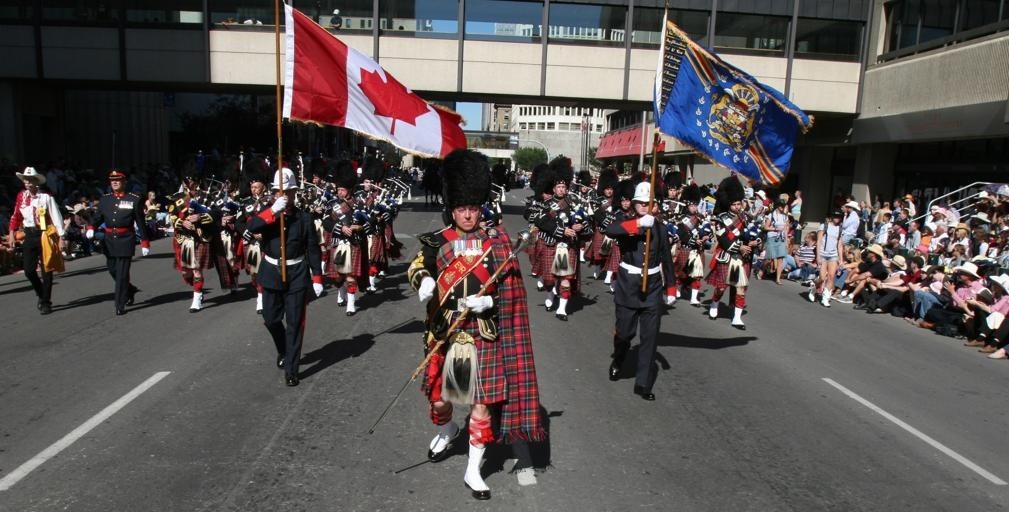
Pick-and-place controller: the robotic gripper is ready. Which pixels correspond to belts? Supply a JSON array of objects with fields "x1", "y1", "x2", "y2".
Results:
[
  {"x1": 105, "y1": 228, "x2": 128, "y2": 233},
  {"x1": 24, "y1": 227, "x2": 39, "y2": 232},
  {"x1": 619, "y1": 261, "x2": 660, "y2": 276},
  {"x1": 265, "y1": 255, "x2": 305, "y2": 266}
]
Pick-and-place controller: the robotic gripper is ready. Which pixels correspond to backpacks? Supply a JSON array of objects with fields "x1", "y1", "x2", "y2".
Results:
[{"x1": 40, "y1": 193, "x2": 65, "y2": 273}]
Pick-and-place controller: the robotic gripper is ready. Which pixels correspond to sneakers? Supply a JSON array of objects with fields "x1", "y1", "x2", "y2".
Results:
[
  {"x1": 837, "y1": 297, "x2": 853, "y2": 303},
  {"x1": 987, "y1": 348, "x2": 1007, "y2": 358},
  {"x1": 830, "y1": 295, "x2": 846, "y2": 300}
]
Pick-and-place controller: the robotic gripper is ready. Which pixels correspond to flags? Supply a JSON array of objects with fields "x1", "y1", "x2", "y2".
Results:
[
  {"x1": 281, "y1": 1, "x2": 466, "y2": 158},
  {"x1": 654, "y1": 16, "x2": 813, "y2": 187}
]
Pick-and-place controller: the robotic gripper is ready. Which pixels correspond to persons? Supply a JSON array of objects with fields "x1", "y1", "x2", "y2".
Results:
[
  {"x1": 839, "y1": 202, "x2": 860, "y2": 268},
  {"x1": 906, "y1": 222, "x2": 919, "y2": 252},
  {"x1": 924, "y1": 261, "x2": 982, "y2": 336},
  {"x1": 987, "y1": 343, "x2": 1009, "y2": 360},
  {"x1": 86, "y1": 171, "x2": 151, "y2": 315},
  {"x1": 791, "y1": 192, "x2": 802, "y2": 227},
  {"x1": 324, "y1": 166, "x2": 370, "y2": 314},
  {"x1": 930, "y1": 226, "x2": 948, "y2": 258},
  {"x1": 246, "y1": 166, "x2": 323, "y2": 385},
  {"x1": 921, "y1": 221, "x2": 935, "y2": 254},
  {"x1": 169, "y1": 155, "x2": 219, "y2": 312},
  {"x1": 810, "y1": 211, "x2": 844, "y2": 309},
  {"x1": 752, "y1": 190, "x2": 790, "y2": 227},
  {"x1": 536, "y1": 155, "x2": 591, "y2": 321},
  {"x1": 51, "y1": 162, "x2": 94, "y2": 261},
  {"x1": 524, "y1": 168, "x2": 707, "y2": 289},
  {"x1": 965, "y1": 183, "x2": 1008, "y2": 272},
  {"x1": 875, "y1": 256, "x2": 924, "y2": 313},
  {"x1": 878, "y1": 214, "x2": 891, "y2": 244},
  {"x1": 604, "y1": 180, "x2": 676, "y2": 401},
  {"x1": 903, "y1": 264, "x2": 951, "y2": 329},
  {"x1": 963, "y1": 274, "x2": 1009, "y2": 354},
  {"x1": 887, "y1": 220, "x2": 905, "y2": 250},
  {"x1": 944, "y1": 224, "x2": 955, "y2": 253},
  {"x1": 925, "y1": 202, "x2": 959, "y2": 229},
  {"x1": 405, "y1": 152, "x2": 542, "y2": 497},
  {"x1": 828, "y1": 242, "x2": 888, "y2": 303},
  {"x1": 669, "y1": 183, "x2": 713, "y2": 305},
  {"x1": 860, "y1": 192, "x2": 915, "y2": 226},
  {"x1": 782, "y1": 234, "x2": 815, "y2": 280},
  {"x1": 211, "y1": 172, "x2": 243, "y2": 296},
  {"x1": 9, "y1": 167, "x2": 66, "y2": 313},
  {"x1": 948, "y1": 246, "x2": 967, "y2": 268},
  {"x1": 757, "y1": 199, "x2": 788, "y2": 287},
  {"x1": 237, "y1": 169, "x2": 269, "y2": 314},
  {"x1": 133, "y1": 188, "x2": 178, "y2": 239},
  {"x1": 947, "y1": 222, "x2": 970, "y2": 259},
  {"x1": 288, "y1": 171, "x2": 409, "y2": 272},
  {"x1": 853, "y1": 254, "x2": 908, "y2": 311},
  {"x1": 705, "y1": 177, "x2": 766, "y2": 327}
]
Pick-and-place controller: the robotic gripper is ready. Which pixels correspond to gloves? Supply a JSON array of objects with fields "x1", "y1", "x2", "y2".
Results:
[
  {"x1": 665, "y1": 296, "x2": 676, "y2": 304},
  {"x1": 313, "y1": 283, "x2": 323, "y2": 296},
  {"x1": 419, "y1": 276, "x2": 436, "y2": 303},
  {"x1": 86, "y1": 229, "x2": 95, "y2": 239},
  {"x1": 142, "y1": 247, "x2": 150, "y2": 256},
  {"x1": 272, "y1": 196, "x2": 288, "y2": 213},
  {"x1": 466, "y1": 294, "x2": 493, "y2": 313},
  {"x1": 637, "y1": 214, "x2": 655, "y2": 228}
]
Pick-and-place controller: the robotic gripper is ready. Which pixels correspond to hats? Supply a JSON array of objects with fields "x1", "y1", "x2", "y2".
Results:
[
  {"x1": 618, "y1": 179, "x2": 635, "y2": 199},
  {"x1": 548, "y1": 155, "x2": 574, "y2": 185},
  {"x1": 989, "y1": 273, "x2": 1009, "y2": 294},
  {"x1": 929, "y1": 280, "x2": 942, "y2": 295},
  {"x1": 830, "y1": 209, "x2": 844, "y2": 217},
  {"x1": 924, "y1": 223, "x2": 937, "y2": 233},
  {"x1": 971, "y1": 212, "x2": 991, "y2": 223},
  {"x1": 971, "y1": 288, "x2": 993, "y2": 302},
  {"x1": 955, "y1": 262, "x2": 981, "y2": 278},
  {"x1": 902, "y1": 194, "x2": 913, "y2": 201},
  {"x1": 845, "y1": 238, "x2": 861, "y2": 246},
  {"x1": 920, "y1": 265, "x2": 931, "y2": 272},
  {"x1": 947, "y1": 221, "x2": 957, "y2": 227},
  {"x1": 16, "y1": 167, "x2": 46, "y2": 184},
  {"x1": 845, "y1": 201, "x2": 860, "y2": 211},
  {"x1": 665, "y1": 172, "x2": 682, "y2": 188},
  {"x1": 250, "y1": 176, "x2": 267, "y2": 186},
  {"x1": 755, "y1": 190, "x2": 767, "y2": 201},
  {"x1": 956, "y1": 223, "x2": 971, "y2": 233},
  {"x1": 926, "y1": 266, "x2": 944, "y2": 274},
  {"x1": 244, "y1": 158, "x2": 274, "y2": 184},
  {"x1": 865, "y1": 244, "x2": 884, "y2": 258},
  {"x1": 632, "y1": 182, "x2": 658, "y2": 202},
  {"x1": 109, "y1": 168, "x2": 128, "y2": 179},
  {"x1": 530, "y1": 163, "x2": 552, "y2": 192},
  {"x1": 974, "y1": 191, "x2": 992, "y2": 199},
  {"x1": 577, "y1": 170, "x2": 592, "y2": 186},
  {"x1": 598, "y1": 169, "x2": 618, "y2": 188},
  {"x1": 682, "y1": 183, "x2": 700, "y2": 203},
  {"x1": 718, "y1": 177, "x2": 744, "y2": 203},
  {"x1": 888, "y1": 255, "x2": 907, "y2": 270},
  {"x1": 366, "y1": 157, "x2": 386, "y2": 183},
  {"x1": 441, "y1": 147, "x2": 492, "y2": 205},
  {"x1": 833, "y1": 210, "x2": 843, "y2": 217},
  {"x1": 271, "y1": 167, "x2": 299, "y2": 190},
  {"x1": 336, "y1": 160, "x2": 357, "y2": 188},
  {"x1": 911, "y1": 256, "x2": 924, "y2": 268},
  {"x1": 775, "y1": 199, "x2": 786, "y2": 207}
]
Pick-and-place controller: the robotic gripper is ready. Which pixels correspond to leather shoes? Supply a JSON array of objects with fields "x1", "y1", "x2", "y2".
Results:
[
  {"x1": 278, "y1": 356, "x2": 286, "y2": 367},
  {"x1": 116, "y1": 305, "x2": 126, "y2": 315},
  {"x1": 125, "y1": 286, "x2": 137, "y2": 306},
  {"x1": 609, "y1": 363, "x2": 621, "y2": 381},
  {"x1": 41, "y1": 304, "x2": 51, "y2": 314},
  {"x1": 633, "y1": 383, "x2": 655, "y2": 401},
  {"x1": 38, "y1": 297, "x2": 42, "y2": 309},
  {"x1": 979, "y1": 345, "x2": 997, "y2": 353},
  {"x1": 854, "y1": 303, "x2": 868, "y2": 309},
  {"x1": 286, "y1": 374, "x2": 298, "y2": 385}
]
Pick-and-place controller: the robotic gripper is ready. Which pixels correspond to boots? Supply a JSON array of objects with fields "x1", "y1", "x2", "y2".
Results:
[
  {"x1": 532, "y1": 267, "x2": 538, "y2": 276},
  {"x1": 366, "y1": 276, "x2": 376, "y2": 292},
  {"x1": 428, "y1": 419, "x2": 461, "y2": 462},
  {"x1": 556, "y1": 298, "x2": 568, "y2": 321},
  {"x1": 256, "y1": 293, "x2": 263, "y2": 311},
  {"x1": 190, "y1": 291, "x2": 203, "y2": 311},
  {"x1": 464, "y1": 442, "x2": 491, "y2": 500},
  {"x1": 593, "y1": 265, "x2": 599, "y2": 278},
  {"x1": 690, "y1": 289, "x2": 700, "y2": 306},
  {"x1": 337, "y1": 286, "x2": 347, "y2": 305},
  {"x1": 346, "y1": 293, "x2": 356, "y2": 315},
  {"x1": 709, "y1": 301, "x2": 719, "y2": 319},
  {"x1": 731, "y1": 307, "x2": 745, "y2": 330},
  {"x1": 821, "y1": 288, "x2": 832, "y2": 306},
  {"x1": 604, "y1": 271, "x2": 613, "y2": 284},
  {"x1": 545, "y1": 286, "x2": 558, "y2": 309},
  {"x1": 537, "y1": 275, "x2": 544, "y2": 289},
  {"x1": 808, "y1": 283, "x2": 816, "y2": 302},
  {"x1": 676, "y1": 284, "x2": 682, "y2": 298},
  {"x1": 580, "y1": 248, "x2": 586, "y2": 262}
]
